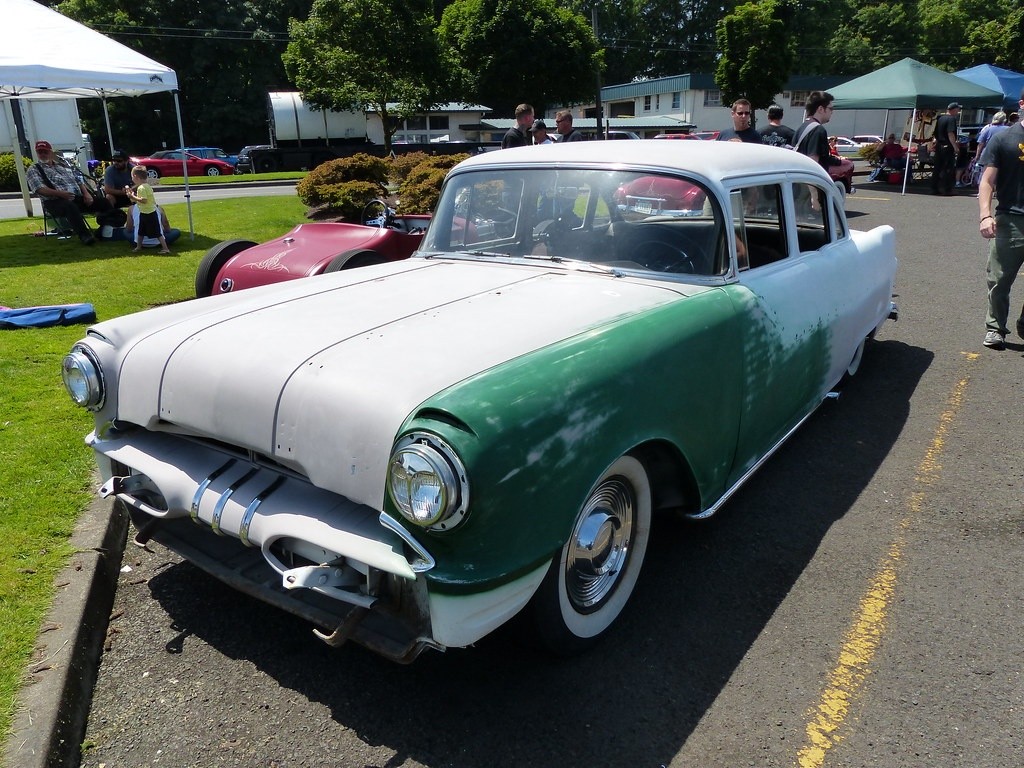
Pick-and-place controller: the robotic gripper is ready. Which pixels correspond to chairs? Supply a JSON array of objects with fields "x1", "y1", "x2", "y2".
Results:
[
  {"x1": 866, "y1": 144, "x2": 935, "y2": 186},
  {"x1": 40, "y1": 163, "x2": 104, "y2": 240}
]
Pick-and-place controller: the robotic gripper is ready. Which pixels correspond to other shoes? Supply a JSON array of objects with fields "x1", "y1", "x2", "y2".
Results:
[
  {"x1": 955, "y1": 183, "x2": 963, "y2": 187},
  {"x1": 158, "y1": 250, "x2": 170, "y2": 255},
  {"x1": 132, "y1": 248, "x2": 144, "y2": 252}
]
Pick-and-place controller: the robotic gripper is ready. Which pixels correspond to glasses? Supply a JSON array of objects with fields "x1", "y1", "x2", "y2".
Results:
[
  {"x1": 824, "y1": 106, "x2": 834, "y2": 111},
  {"x1": 889, "y1": 137, "x2": 894, "y2": 139},
  {"x1": 556, "y1": 119, "x2": 565, "y2": 125},
  {"x1": 734, "y1": 111, "x2": 751, "y2": 117},
  {"x1": 112, "y1": 158, "x2": 124, "y2": 163}
]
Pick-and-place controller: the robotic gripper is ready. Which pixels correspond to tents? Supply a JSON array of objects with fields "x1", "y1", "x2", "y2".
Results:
[
  {"x1": 0, "y1": 0, "x2": 197, "y2": 242},
  {"x1": 802, "y1": 57, "x2": 1005, "y2": 196},
  {"x1": 950, "y1": 65, "x2": 1024, "y2": 99}
]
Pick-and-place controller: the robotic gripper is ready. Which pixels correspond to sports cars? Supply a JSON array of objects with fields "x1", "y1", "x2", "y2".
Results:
[{"x1": 195, "y1": 198, "x2": 478, "y2": 302}]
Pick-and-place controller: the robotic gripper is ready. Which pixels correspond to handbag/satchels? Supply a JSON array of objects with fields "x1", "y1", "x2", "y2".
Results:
[
  {"x1": 889, "y1": 169, "x2": 902, "y2": 183},
  {"x1": 868, "y1": 167, "x2": 883, "y2": 181}
]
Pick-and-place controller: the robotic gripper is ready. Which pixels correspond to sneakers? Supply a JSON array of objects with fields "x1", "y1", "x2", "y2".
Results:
[
  {"x1": 983, "y1": 331, "x2": 1005, "y2": 347},
  {"x1": 80, "y1": 231, "x2": 94, "y2": 245}
]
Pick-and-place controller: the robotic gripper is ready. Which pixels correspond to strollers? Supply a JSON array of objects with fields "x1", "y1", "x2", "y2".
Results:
[{"x1": 960, "y1": 156, "x2": 985, "y2": 189}]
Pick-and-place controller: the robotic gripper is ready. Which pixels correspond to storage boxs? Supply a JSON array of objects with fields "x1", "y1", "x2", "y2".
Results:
[{"x1": 887, "y1": 171, "x2": 902, "y2": 185}]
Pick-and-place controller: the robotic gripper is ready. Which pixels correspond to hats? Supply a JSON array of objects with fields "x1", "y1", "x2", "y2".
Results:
[
  {"x1": 960, "y1": 137, "x2": 972, "y2": 143},
  {"x1": 35, "y1": 141, "x2": 52, "y2": 151},
  {"x1": 947, "y1": 102, "x2": 963, "y2": 109},
  {"x1": 112, "y1": 149, "x2": 128, "y2": 160},
  {"x1": 528, "y1": 121, "x2": 546, "y2": 131}
]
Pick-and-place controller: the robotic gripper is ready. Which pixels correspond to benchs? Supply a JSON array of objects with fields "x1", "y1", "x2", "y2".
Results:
[{"x1": 602, "y1": 219, "x2": 825, "y2": 266}]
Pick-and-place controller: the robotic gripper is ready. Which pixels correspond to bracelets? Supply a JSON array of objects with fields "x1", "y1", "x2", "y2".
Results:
[{"x1": 980, "y1": 215, "x2": 992, "y2": 223}]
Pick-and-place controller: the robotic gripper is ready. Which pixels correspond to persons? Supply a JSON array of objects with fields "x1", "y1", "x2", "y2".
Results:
[
  {"x1": 104, "y1": 150, "x2": 181, "y2": 254},
  {"x1": 502, "y1": 104, "x2": 535, "y2": 225},
  {"x1": 529, "y1": 121, "x2": 556, "y2": 220},
  {"x1": 932, "y1": 101, "x2": 963, "y2": 195},
  {"x1": 556, "y1": 110, "x2": 584, "y2": 215},
  {"x1": 716, "y1": 99, "x2": 762, "y2": 144},
  {"x1": 753, "y1": 104, "x2": 795, "y2": 149},
  {"x1": 879, "y1": 133, "x2": 917, "y2": 186},
  {"x1": 826, "y1": 135, "x2": 839, "y2": 156},
  {"x1": 27, "y1": 141, "x2": 127, "y2": 245},
  {"x1": 978, "y1": 93, "x2": 1024, "y2": 347},
  {"x1": 792, "y1": 91, "x2": 835, "y2": 225},
  {"x1": 972, "y1": 111, "x2": 1019, "y2": 188}
]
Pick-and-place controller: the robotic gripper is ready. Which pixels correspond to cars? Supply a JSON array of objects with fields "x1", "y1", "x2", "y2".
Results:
[
  {"x1": 129, "y1": 148, "x2": 234, "y2": 179},
  {"x1": 530, "y1": 131, "x2": 890, "y2": 219},
  {"x1": 56, "y1": 136, "x2": 904, "y2": 675},
  {"x1": 171, "y1": 143, "x2": 272, "y2": 176}
]
